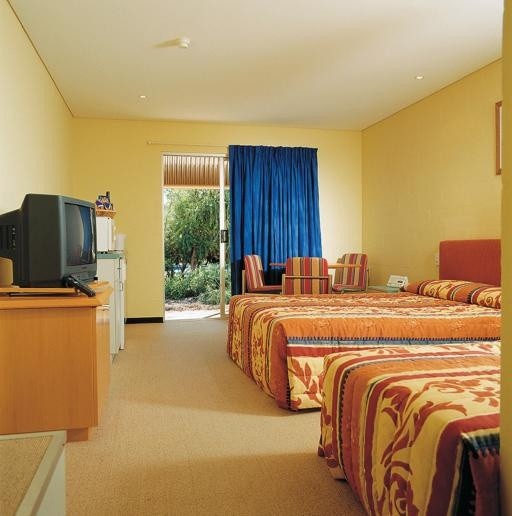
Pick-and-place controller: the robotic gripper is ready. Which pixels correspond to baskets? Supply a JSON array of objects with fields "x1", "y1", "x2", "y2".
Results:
[{"x1": 96, "y1": 210, "x2": 117, "y2": 219}]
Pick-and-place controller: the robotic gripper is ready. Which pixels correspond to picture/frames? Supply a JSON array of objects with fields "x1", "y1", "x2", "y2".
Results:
[{"x1": 495, "y1": 100, "x2": 503, "y2": 175}]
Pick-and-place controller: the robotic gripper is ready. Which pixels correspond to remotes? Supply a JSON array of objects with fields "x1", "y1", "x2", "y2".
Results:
[{"x1": 68, "y1": 273, "x2": 96, "y2": 297}]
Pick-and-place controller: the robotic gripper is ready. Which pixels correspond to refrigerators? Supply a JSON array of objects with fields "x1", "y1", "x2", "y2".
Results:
[{"x1": 97, "y1": 253, "x2": 126, "y2": 355}]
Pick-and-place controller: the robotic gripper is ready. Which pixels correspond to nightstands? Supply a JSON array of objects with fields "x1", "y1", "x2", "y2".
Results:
[{"x1": 367, "y1": 285, "x2": 403, "y2": 293}]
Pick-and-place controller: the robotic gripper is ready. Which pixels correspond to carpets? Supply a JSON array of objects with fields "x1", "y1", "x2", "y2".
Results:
[{"x1": 68, "y1": 319, "x2": 369, "y2": 515}]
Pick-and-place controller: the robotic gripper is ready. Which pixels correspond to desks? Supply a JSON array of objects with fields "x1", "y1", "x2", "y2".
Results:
[{"x1": 269, "y1": 263, "x2": 363, "y2": 269}]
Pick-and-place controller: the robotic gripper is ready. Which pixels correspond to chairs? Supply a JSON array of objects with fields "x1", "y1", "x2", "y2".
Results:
[
  {"x1": 280, "y1": 257, "x2": 332, "y2": 295},
  {"x1": 241, "y1": 255, "x2": 282, "y2": 294},
  {"x1": 331, "y1": 252, "x2": 369, "y2": 294}
]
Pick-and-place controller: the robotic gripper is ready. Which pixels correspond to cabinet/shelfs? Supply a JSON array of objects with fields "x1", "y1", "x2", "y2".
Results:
[{"x1": 1, "y1": 279, "x2": 114, "y2": 445}]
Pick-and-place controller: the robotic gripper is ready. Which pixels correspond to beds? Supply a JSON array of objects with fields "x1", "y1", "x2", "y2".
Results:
[
  {"x1": 225, "y1": 238, "x2": 501, "y2": 412},
  {"x1": 318, "y1": 341, "x2": 500, "y2": 514}
]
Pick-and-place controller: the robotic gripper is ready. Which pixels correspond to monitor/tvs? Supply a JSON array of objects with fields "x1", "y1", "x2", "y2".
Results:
[{"x1": 0, "y1": 193, "x2": 97, "y2": 288}]
